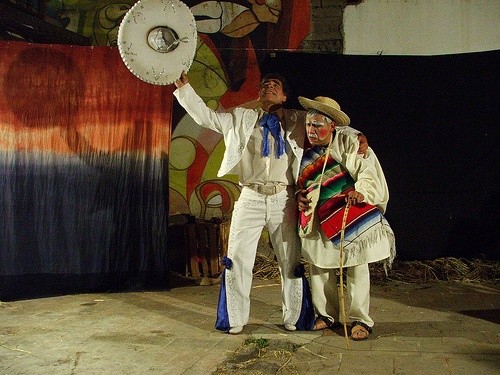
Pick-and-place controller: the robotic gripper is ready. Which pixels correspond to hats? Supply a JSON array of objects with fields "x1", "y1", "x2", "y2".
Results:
[
  {"x1": 298, "y1": 95, "x2": 350, "y2": 127},
  {"x1": 117, "y1": 0, "x2": 197, "y2": 86}
]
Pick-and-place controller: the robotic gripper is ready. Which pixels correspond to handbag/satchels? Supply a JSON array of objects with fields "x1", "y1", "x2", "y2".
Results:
[{"x1": 298, "y1": 182, "x2": 321, "y2": 238}]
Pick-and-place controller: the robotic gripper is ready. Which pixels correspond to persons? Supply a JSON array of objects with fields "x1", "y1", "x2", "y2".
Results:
[
  {"x1": 293, "y1": 97, "x2": 395, "y2": 342},
  {"x1": 175, "y1": 69, "x2": 369, "y2": 334}
]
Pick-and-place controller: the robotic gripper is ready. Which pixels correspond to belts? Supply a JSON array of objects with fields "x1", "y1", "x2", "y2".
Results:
[{"x1": 247, "y1": 183, "x2": 286, "y2": 195}]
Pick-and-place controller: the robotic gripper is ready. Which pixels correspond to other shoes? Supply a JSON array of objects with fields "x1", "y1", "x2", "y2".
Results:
[
  {"x1": 312, "y1": 315, "x2": 341, "y2": 331},
  {"x1": 229, "y1": 326, "x2": 243, "y2": 333},
  {"x1": 285, "y1": 323, "x2": 296, "y2": 330},
  {"x1": 350, "y1": 321, "x2": 372, "y2": 340}
]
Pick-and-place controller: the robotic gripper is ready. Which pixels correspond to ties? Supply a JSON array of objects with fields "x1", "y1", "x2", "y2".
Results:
[{"x1": 259, "y1": 113, "x2": 283, "y2": 159}]
